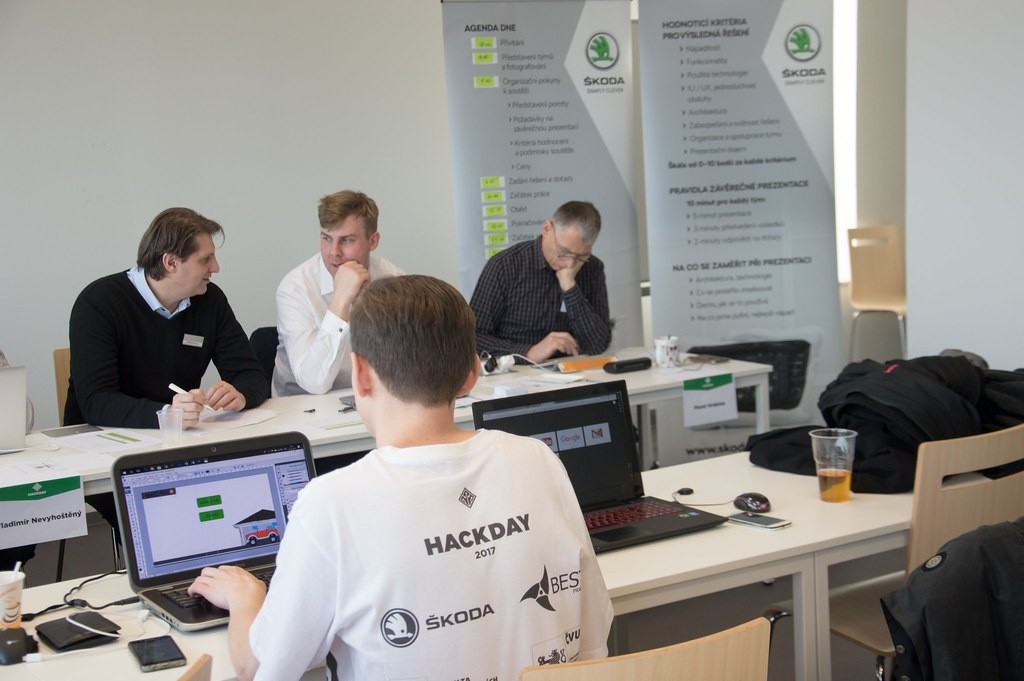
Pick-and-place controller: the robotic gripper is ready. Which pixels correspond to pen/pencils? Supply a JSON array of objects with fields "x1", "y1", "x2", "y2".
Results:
[{"x1": 168, "y1": 383, "x2": 215, "y2": 412}]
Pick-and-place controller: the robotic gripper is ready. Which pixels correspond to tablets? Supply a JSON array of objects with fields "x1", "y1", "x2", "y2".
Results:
[{"x1": 532, "y1": 354, "x2": 586, "y2": 368}]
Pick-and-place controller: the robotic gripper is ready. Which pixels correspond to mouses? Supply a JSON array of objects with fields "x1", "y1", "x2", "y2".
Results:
[{"x1": 733, "y1": 493, "x2": 770, "y2": 513}]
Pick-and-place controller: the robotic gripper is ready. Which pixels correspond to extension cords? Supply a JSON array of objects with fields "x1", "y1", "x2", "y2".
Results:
[{"x1": 478, "y1": 360, "x2": 509, "y2": 376}]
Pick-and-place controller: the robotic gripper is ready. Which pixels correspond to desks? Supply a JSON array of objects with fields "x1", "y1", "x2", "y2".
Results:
[
  {"x1": 0, "y1": 346, "x2": 773, "y2": 596},
  {"x1": 2, "y1": 446, "x2": 994, "y2": 681}
]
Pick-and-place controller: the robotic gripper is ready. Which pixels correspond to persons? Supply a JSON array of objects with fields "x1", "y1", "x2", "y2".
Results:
[
  {"x1": 469, "y1": 200, "x2": 613, "y2": 364},
  {"x1": 271, "y1": 189, "x2": 407, "y2": 402},
  {"x1": 187, "y1": 275, "x2": 615, "y2": 681},
  {"x1": 63, "y1": 207, "x2": 270, "y2": 430}
]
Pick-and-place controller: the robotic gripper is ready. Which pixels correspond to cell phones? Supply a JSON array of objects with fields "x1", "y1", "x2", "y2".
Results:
[
  {"x1": 339, "y1": 395, "x2": 356, "y2": 406},
  {"x1": 128, "y1": 634, "x2": 187, "y2": 673},
  {"x1": 728, "y1": 512, "x2": 791, "y2": 527}
]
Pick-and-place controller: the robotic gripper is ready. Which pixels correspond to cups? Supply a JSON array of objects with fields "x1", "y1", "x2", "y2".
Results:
[
  {"x1": 809, "y1": 429, "x2": 858, "y2": 503},
  {"x1": 0, "y1": 571, "x2": 25, "y2": 630},
  {"x1": 654, "y1": 336, "x2": 679, "y2": 367},
  {"x1": 157, "y1": 409, "x2": 184, "y2": 442}
]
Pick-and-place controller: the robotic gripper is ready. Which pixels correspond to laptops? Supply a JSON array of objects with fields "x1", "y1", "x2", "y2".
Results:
[
  {"x1": 113, "y1": 430, "x2": 316, "y2": 631},
  {"x1": 472, "y1": 379, "x2": 728, "y2": 554},
  {"x1": 0, "y1": 365, "x2": 27, "y2": 450}
]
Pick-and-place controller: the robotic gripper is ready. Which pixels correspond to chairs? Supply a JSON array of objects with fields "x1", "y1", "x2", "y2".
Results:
[
  {"x1": 763, "y1": 425, "x2": 1024, "y2": 681},
  {"x1": 515, "y1": 607, "x2": 771, "y2": 681},
  {"x1": 848, "y1": 218, "x2": 906, "y2": 365}
]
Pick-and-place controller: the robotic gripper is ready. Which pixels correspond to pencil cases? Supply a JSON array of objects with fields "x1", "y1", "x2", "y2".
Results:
[{"x1": 603, "y1": 357, "x2": 651, "y2": 374}]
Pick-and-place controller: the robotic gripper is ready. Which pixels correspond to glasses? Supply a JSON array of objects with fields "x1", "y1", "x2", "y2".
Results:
[{"x1": 552, "y1": 227, "x2": 592, "y2": 262}]
